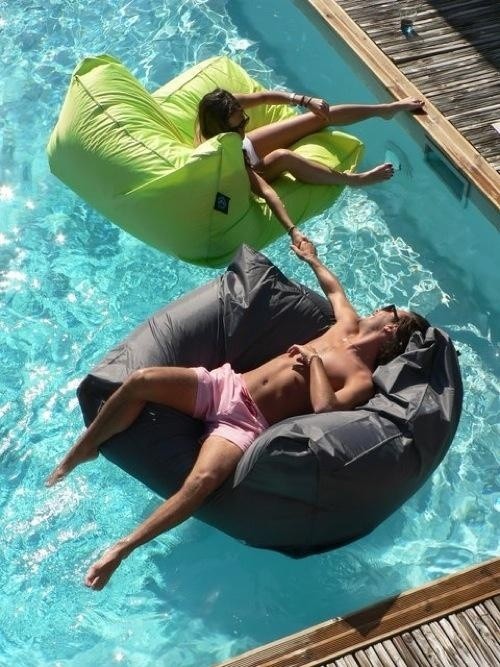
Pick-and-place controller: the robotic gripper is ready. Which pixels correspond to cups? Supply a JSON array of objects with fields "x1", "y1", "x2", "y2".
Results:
[{"x1": 399, "y1": 7, "x2": 418, "y2": 34}]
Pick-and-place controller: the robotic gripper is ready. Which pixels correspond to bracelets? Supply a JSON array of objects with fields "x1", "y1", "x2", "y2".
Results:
[
  {"x1": 288, "y1": 225, "x2": 295, "y2": 235},
  {"x1": 300, "y1": 95, "x2": 306, "y2": 107},
  {"x1": 305, "y1": 96, "x2": 313, "y2": 106},
  {"x1": 289, "y1": 93, "x2": 296, "y2": 107}
]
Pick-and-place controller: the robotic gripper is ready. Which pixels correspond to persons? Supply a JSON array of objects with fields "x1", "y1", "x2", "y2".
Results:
[
  {"x1": 194, "y1": 88, "x2": 425, "y2": 249},
  {"x1": 43, "y1": 239, "x2": 420, "y2": 591}
]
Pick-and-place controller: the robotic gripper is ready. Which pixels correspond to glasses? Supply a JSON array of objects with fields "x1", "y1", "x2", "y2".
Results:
[
  {"x1": 229, "y1": 110, "x2": 251, "y2": 133},
  {"x1": 382, "y1": 303, "x2": 398, "y2": 320}
]
